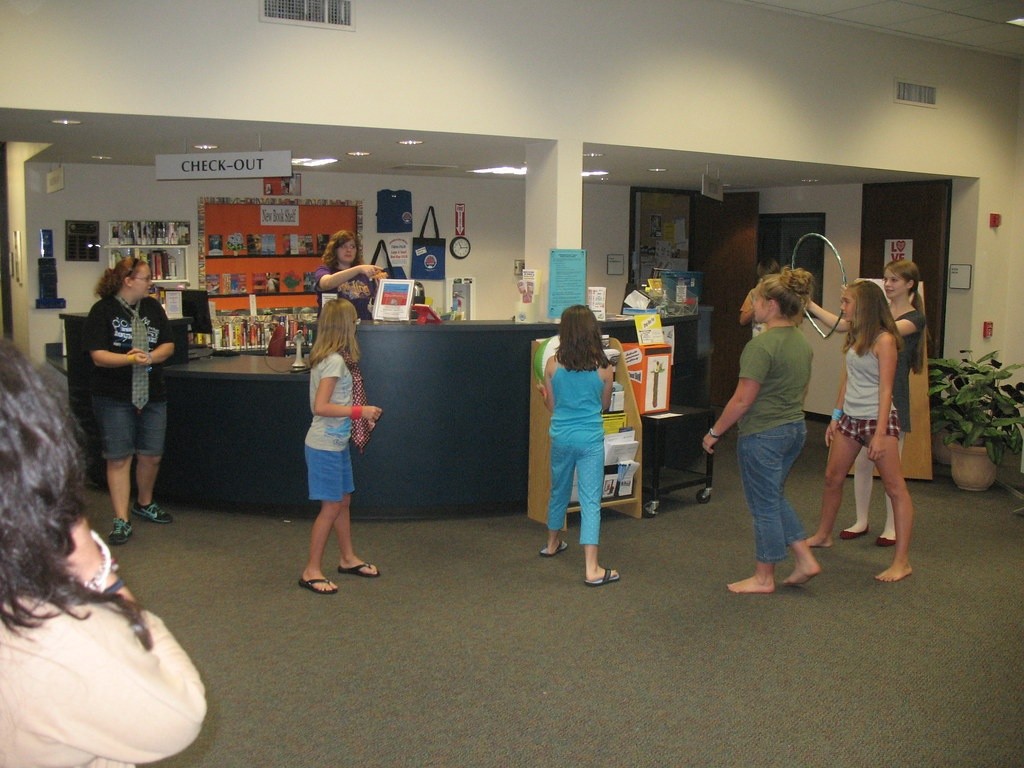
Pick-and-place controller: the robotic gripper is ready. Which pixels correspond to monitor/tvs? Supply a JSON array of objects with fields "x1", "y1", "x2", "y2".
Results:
[{"x1": 164, "y1": 287, "x2": 213, "y2": 335}]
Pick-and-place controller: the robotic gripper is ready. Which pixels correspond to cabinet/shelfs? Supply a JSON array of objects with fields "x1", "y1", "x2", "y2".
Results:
[
  {"x1": 527, "y1": 334, "x2": 643, "y2": 530},
  {"x1": 205, "y1": 254, "x2": 325, "y2": 299},
  {"x1": 103, "y1": 243, "x2": 189, "y2": 285}
]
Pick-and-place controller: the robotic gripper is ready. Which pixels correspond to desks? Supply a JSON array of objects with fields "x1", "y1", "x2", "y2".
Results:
[{"x1": 639, "y1": 406, "x2": 714, "y2": 517}]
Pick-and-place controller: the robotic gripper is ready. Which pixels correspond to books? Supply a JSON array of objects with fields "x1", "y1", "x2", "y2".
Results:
[
  {"x1": 111, "y1": 248, "x2": 171, "y2": 280},
  {"x1": 188, "y1": 316, "x2": 318, "y2": 348},
  {"x1": 108, "y1": 220, "x2": 190, "y2": 245}
]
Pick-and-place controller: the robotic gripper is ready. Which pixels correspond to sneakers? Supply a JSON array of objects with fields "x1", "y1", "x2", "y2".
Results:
[
  {"x1": 108, "y1": 518, "x2": 133, "y2": 544},
  {"x1": 130, "y1": 502, "x2": 172, "y2": 524}
]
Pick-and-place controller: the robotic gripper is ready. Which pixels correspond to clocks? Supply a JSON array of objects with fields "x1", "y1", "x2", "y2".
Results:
[{"x1": 450, "y1": 237, "x2": 470, "y2": 259}]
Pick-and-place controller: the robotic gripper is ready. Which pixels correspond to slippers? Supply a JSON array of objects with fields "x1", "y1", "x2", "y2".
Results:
[
  {"x1": 337, "y1": 562, "x2": 381, "y2": 578},
  {"x1": 539, "y1": 541, "x2": 567, "y2": 556},
  {"x1": 586, "y1": 568, "x2": 619, "y2": 586},
  {"x1": 299, "y1": 575, "x2": 338, "y2": 594}
]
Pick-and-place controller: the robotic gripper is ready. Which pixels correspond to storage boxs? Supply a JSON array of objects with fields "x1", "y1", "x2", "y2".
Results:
[{"x1": 661, "y1": 271, "x2": 705, "y2": 304}]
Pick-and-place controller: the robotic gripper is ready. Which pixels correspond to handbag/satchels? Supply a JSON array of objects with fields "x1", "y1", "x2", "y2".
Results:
[
  {"x1": 410, "y1": 206, "x2": 446, "y2": 280},
  {"x1": 367, "y1": 240, "x2": 406, "y2": 296}
]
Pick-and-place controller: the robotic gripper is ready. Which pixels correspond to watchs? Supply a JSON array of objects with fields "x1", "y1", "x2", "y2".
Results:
[{"x1": 708, "y1": 428, "x2": 719, "y2": 439}]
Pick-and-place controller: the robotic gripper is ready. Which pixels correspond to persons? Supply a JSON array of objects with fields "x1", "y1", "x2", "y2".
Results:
[
  {"x1": 800, "y1": 280, "x2": 914, "y2": 583},
  {"x1": 537, "y1": 304, "x2": 619, "y2": 587},
  {"x1": 0, "y1": 337, "x2": 208, "y2": 768},
  {"x1": 313, "y1": 229, "x2": 383, "y2": 321},
  {"x1": 702, "y1": 257, "x2": 821, "y2": 593},
  {"x1": 298, "y1": 298, "x2": 382, "y2": 592},
  {"x1": 804, "y1": 259, "x2": 926, "y2": 548},
  {"x1": 79, "y1": 256, "x2": 175, "y2": 545}
]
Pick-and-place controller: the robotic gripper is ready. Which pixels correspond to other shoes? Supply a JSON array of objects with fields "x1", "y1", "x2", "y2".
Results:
[
  {"x1": 876, "y1": 535, "x2": 896, "y2": 547},
  {"x1": 839, "y1": 523, "x2": 868, "y2": 539}
]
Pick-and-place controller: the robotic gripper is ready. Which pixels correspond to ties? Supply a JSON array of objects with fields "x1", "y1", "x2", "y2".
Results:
[{"x1": 115, "y1": 294, "x2": 149, "y2": 409}]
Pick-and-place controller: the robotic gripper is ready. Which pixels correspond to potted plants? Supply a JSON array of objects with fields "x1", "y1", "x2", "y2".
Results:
[{"x1": 925, "y1": 348, "x2": 1024, "y2": 493}]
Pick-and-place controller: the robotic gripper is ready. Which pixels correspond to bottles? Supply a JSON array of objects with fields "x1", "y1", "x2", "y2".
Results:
[{"x1": 212, "y1": 313, "x2": 313, "y2": 349}]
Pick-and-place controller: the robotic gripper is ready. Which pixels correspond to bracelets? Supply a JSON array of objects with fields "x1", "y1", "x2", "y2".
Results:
[
  {"x1": 90, "y1": 526, "x2": 118, "y2": 593},
  {"x1": 350, "y1": 405, "x2": 362, "y2": 421},
  {"x1": 101, "y1": 578, "x2": 126, "y2": 596},
  {"x1": 146, "y1": 353, "x2": 152, "y2": 365},
  {"x1": 832, "y1": 409, "x2": 843, "y2": 422},
  {"x1": 127, "y1": 354, "x2": 135, "y2": 364}
]
